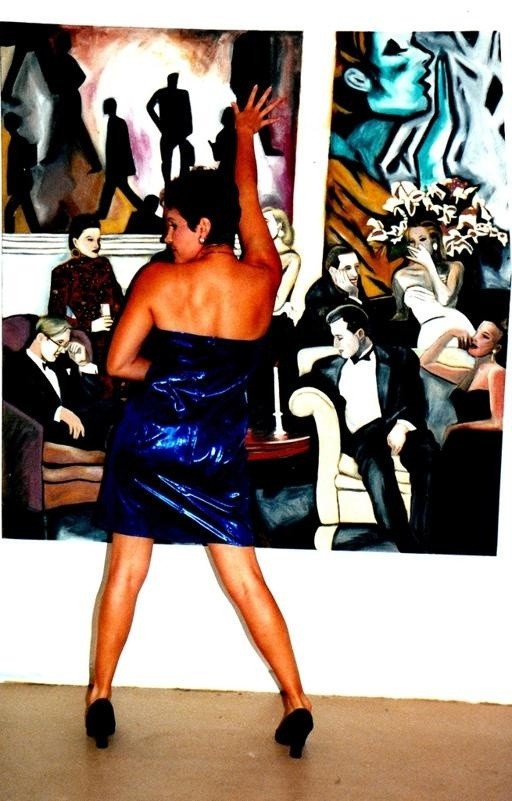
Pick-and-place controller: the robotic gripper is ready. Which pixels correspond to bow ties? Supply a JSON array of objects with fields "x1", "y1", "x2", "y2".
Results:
[{"x1": 42, "y1": 363, "x2": 53, "y2": 370}]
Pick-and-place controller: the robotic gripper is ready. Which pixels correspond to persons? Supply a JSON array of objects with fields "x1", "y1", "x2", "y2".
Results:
[{"x1": 84, "y1": 83, "x2": 315, "y2": 758}]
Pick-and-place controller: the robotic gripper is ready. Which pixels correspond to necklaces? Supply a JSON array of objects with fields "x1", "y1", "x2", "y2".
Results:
[{"x1": 200, "y1": 251, "x2": 237, "y2": 259}]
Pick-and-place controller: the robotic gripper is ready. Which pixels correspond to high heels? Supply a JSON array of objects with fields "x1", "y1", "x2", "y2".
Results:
[
  {"x1": 275, "y1": 708, "x2": 314, "y2": 759},
  {"x1": 85, "y1": 698, "x2": 116, "y2": 749}
]
